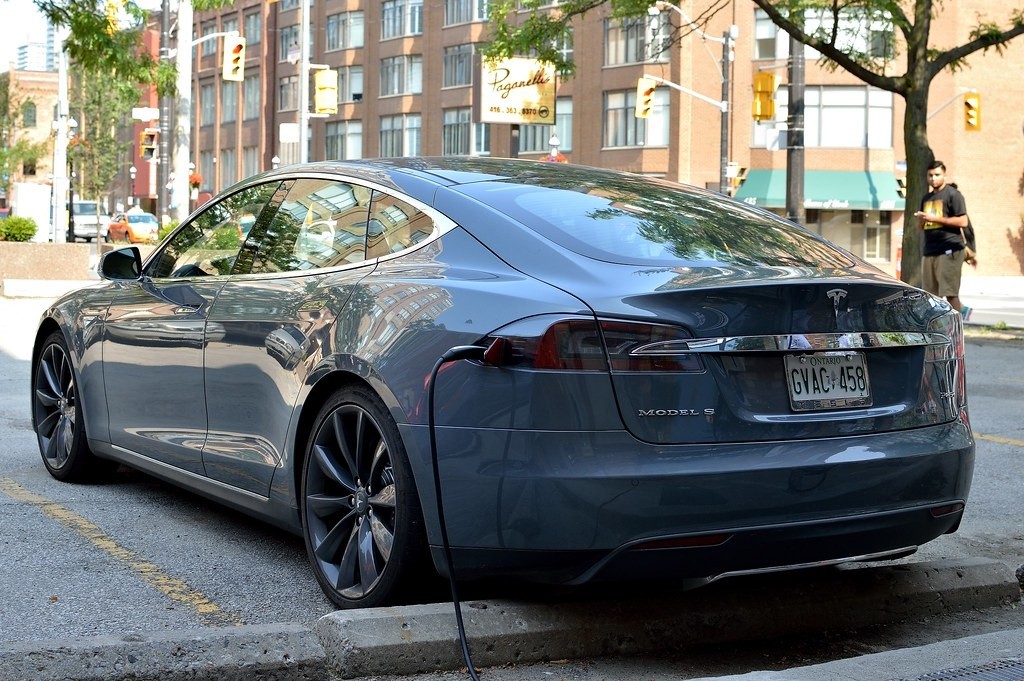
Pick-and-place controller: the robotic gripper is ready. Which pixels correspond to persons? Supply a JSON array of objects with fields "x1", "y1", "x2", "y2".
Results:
[{"x1": 914, "y1": 161, "x2": 977, "y2": 321}]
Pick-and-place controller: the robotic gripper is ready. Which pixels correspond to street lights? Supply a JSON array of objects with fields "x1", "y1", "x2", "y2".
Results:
[{"x1": 647, "y1": 0, "x2": 730, "y2": 195}]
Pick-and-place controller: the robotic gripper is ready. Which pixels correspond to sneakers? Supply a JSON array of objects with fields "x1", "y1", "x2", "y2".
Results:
[{"x1": 961, "y1": 306, "x2": 972, "y2": 321}]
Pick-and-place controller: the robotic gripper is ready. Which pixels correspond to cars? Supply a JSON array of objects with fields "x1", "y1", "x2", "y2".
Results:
[
  {"x1": 106, "y1": 206, "x2": 158, "y2": 244},
  {"x1": 0, "y1": 206, "x2": 53, "y2": 241},
  {"x1": 28, "y1": 155, "x2": 976, "y2": 612}
]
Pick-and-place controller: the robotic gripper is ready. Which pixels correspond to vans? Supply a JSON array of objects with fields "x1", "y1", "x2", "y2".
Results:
[{"x1": 65, "y1": 200, "x2": 112, "y2": 241}]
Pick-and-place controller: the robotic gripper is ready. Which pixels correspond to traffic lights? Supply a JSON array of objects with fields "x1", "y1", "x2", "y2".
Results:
[
  {"x1": 222, "y1": 36, "x2": 247, "y2": 82},
  {"x1": 140, "y1": 132, "x2": 156, "y2": 159},
  {"x1": 635, "y1": 78, "x2": 656, "y2": 119},
  {"x1": 964, "y1": 94, "x2": 982, "y2": 132}
]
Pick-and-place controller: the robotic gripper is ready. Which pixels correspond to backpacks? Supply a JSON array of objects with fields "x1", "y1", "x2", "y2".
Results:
[{"x1": 958, "y1": 214, "x2": 976, "y2": 260}]
